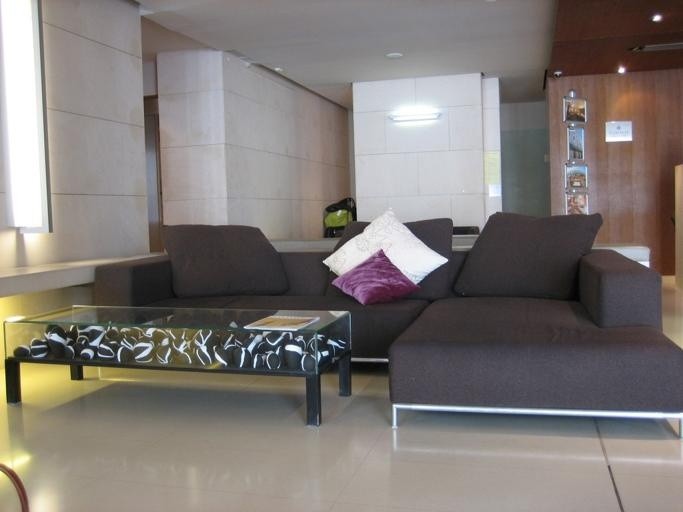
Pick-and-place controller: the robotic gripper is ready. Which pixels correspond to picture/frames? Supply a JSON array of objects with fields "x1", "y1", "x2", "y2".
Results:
[{"x1": 562, "y1": 97, "x2": 589, "y2": 215}]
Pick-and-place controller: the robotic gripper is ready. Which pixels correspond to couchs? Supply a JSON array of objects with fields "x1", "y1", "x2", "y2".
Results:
[{"x1": 94, "y1": 251, "x2": 466, "y2": 439}]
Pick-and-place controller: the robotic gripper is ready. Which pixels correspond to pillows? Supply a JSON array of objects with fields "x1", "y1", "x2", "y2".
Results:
[
  {"x1": 326, "y1": 219, "x2": 455, "y2": 299},
  {"x1": 318, "y1": 207, "x2": 447, "y2": 287},
  {"x1": 331, "y1": 247, "x2": 420, "y2": 304},
  {"x1": 454, "y1": 212, "x2": 603, "y2": 298},
  {"x1": 160, "y1": 224, "x2": 287, "y2": 297}
]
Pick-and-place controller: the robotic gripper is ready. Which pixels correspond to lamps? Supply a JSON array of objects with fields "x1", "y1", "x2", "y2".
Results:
[{"x1": 390, "y1": 114, "x2": 440, "y2": 123}]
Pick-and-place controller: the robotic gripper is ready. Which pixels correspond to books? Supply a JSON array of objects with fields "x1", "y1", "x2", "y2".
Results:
[{"x1": 243, "y1": 316, "x2": 321, "y2": 332}]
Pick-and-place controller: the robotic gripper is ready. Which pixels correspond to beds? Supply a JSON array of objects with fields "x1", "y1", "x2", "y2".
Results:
[{"x1": 388, "y1": 246, "x2": 683, "y2": 438}]
